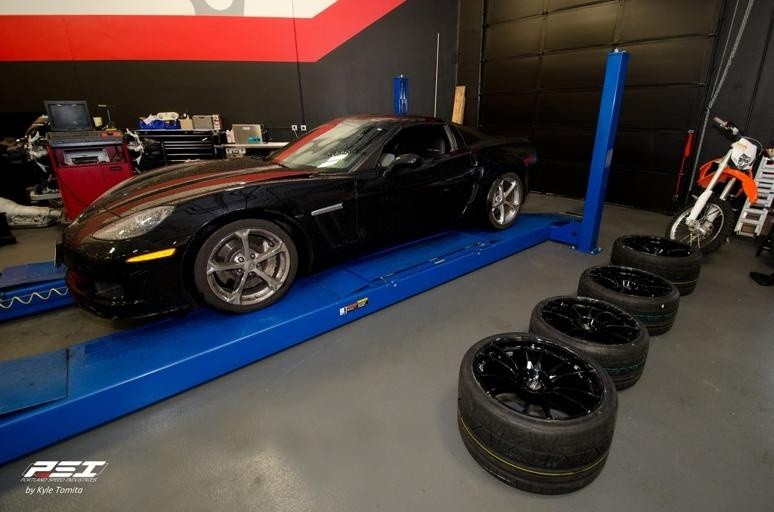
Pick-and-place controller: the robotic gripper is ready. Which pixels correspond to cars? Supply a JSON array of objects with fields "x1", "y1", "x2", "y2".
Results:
[{"x1": 53, "y1": 116, "x2": 537, "y2": 328}]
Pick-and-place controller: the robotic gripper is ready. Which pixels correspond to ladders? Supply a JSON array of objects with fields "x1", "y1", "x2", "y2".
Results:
[{"x1": 732, "y1": 156, "x2": 774, "y2": 243}]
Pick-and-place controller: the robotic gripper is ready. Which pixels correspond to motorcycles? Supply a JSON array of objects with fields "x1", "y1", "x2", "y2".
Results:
[
  {"x1": 15, "y1": 117, "x2": 143, "y2": 208},
  {"x1": 666, "y1": 116, "x2": 773, "y2": 253}
]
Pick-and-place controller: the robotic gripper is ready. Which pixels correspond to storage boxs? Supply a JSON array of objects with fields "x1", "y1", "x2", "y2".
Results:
[
  {"x1": 232, "y1": 123, "x2": 261, "y2": 144},
  {"x1": 179, "y1": 118, "x2": 193, "y2": 129},
  {"x1": 192, "y1": 115, "x2": 212, "y2": 129}
]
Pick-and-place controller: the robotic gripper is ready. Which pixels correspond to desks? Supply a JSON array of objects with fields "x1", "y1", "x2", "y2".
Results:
[{"x1": 224, "y1": 141, "x2": 290, "y2": 161}]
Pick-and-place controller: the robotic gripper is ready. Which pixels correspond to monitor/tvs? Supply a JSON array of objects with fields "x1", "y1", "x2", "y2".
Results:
[{"x1": 44, "y1": 100, "x2": 93, "y2": 132}]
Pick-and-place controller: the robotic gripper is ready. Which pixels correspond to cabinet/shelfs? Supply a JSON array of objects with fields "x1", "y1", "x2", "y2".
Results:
[{"x1": 48, "y1": 140, "x2": 133, "y2": 222}]
